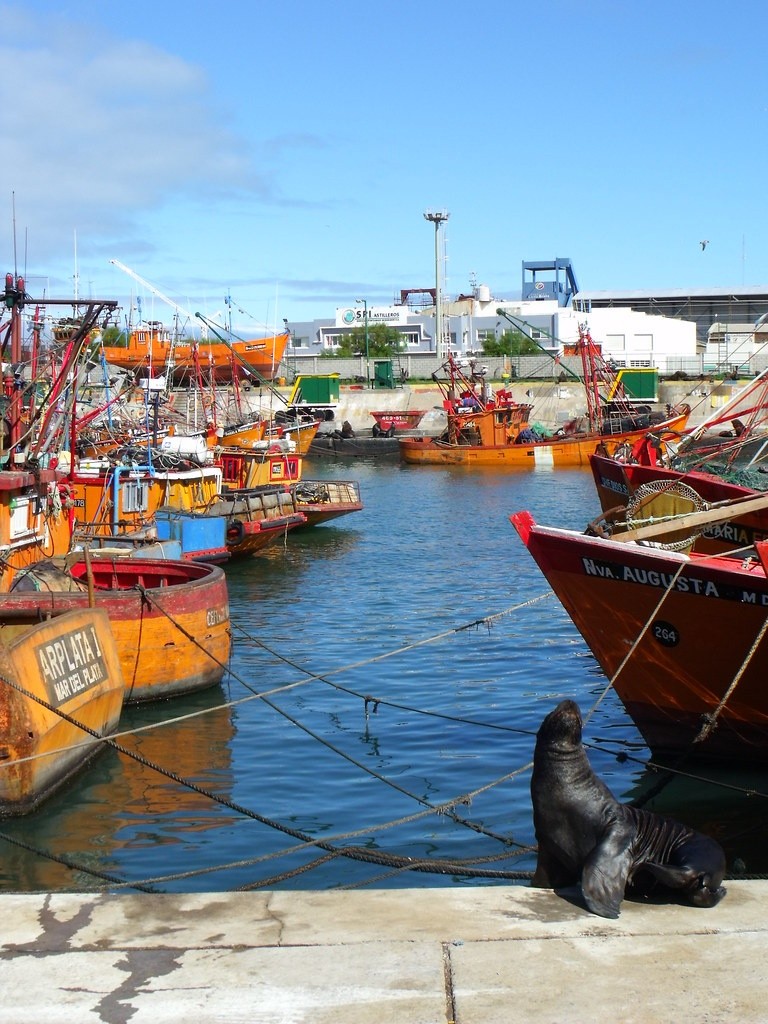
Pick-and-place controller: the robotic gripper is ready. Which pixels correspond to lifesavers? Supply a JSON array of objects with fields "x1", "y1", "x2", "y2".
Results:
[
  {"x1": 137, "y1": 409, "x2": 145, "y2": 418},
  {"x1": 225, "y1": 519, "x2": 245, "y2": 545},
  {"x1": 495, "y1": 396, "x2": 499, "y2": 407},
  {"x1": 202, "y1": 394, "x2": 213, "y2": 407},
  {"x1": 186, "y1": 398, "x2": 198, "y2": 411}
]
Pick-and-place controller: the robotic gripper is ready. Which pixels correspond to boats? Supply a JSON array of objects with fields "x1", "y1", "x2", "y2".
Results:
[
  {"x1": 507, "y1": 491, "x2": 768, "y2": 767},
  {"x1": 370, "y1": 409, "x2": 430, "y2": 438},
  {"x1": 392, "y1": 309, "x2": 691, "y2": 469},
  {"x1": 587, "y1": 314, "x2": 767, "y2": 562}
]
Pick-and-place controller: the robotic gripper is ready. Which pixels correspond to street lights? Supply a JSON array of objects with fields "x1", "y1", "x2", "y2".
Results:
[{"x1": 0, "y1": 185, "x2": 364, "y2": 819}]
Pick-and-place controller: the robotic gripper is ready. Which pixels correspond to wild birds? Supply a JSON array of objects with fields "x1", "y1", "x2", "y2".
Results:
[{"x1": 700, "y1": 240, "x2": 709, "y2": 250}]
